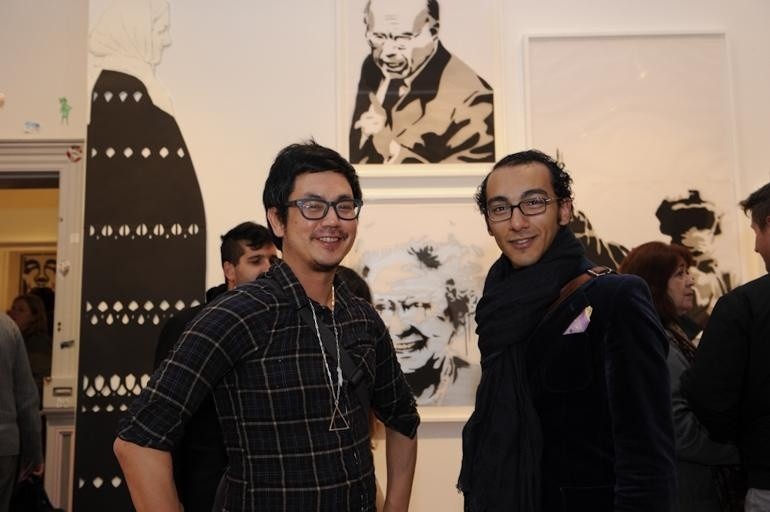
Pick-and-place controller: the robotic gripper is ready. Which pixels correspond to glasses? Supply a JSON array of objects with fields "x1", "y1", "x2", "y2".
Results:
[
  {"x1": 486, "y1": 196, "x2": 562, "y2": 222},
  {"x1": 284, "y1": 198, "x2": 363, "y2": 220}
]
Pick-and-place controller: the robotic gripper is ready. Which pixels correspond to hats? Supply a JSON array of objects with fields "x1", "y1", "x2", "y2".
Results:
[{"x1": 655, "y1": 191, "x2": 723, "y2": 232}]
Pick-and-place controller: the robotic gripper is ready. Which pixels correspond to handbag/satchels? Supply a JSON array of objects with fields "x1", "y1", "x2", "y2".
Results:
[{"x1": 10, "y1": 481, "x2": 57, "y2": 510}]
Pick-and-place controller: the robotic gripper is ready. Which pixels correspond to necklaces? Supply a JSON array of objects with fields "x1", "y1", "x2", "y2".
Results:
[{"x1": 302, "y1": 286, "x2": 352, "y2": 435}]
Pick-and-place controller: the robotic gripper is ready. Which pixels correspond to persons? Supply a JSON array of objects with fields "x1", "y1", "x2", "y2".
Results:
[
  {"x1": 347, "y1": 1, "x2": 496, "y2": 165},
  {"x1": 674, "y1": 245, "x2": 746, "y2": 346},
  {"x1": 6, "y1": 295, "x2": 52, "y2": 387},
  {"x1": 616, "y1": 242, "x2": 749, "y2": 512},
  {"x1": 0, "y1": 308, "x2": 44, "y2": 511},
  {"x1": 454, "y1": 147, "x2": 673, "y2": 512},
  {"x1": 152, "y1": 220, "x2": 280, "y2": 510},
  {"x1": 689, "y1": 182, "x2": 770, "y2": 512},
  {"x1": 112, "y1": 137, "x2": 421, "y2": 512}
]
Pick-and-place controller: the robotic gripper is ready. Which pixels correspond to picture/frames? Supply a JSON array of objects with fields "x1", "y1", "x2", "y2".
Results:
[
  {"x1": 338, "y1": 186, "x2": 503, "y2": 421},
  {"x1": 338, "y1": 1, "x2": 503, "y2": 178},
  {"x1": 522, "y1": 25, "x2": 748, "y2": 345}
]
